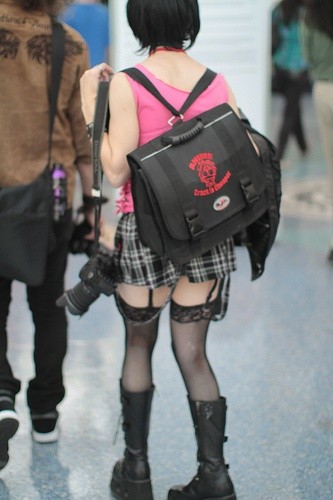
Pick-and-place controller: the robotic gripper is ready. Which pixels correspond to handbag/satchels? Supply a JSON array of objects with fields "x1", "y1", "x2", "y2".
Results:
[{"x1": 0, "y1": 165, "x2": 57, "y2": 288}]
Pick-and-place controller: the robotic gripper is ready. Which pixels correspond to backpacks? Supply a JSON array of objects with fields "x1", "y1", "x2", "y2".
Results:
[{"x1": 121, "y1": 68, "x2": 267, "y2": 264}]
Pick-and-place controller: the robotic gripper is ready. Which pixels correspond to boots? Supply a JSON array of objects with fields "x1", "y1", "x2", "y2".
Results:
[
  {"x1": 109, "y1": 380, "x2": 153, "y2": 500},
  {"x1": 167, "y1": 396, "x2": 237, "y2": 500}
]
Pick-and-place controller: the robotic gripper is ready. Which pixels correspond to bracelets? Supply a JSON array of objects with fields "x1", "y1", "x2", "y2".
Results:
[{"x1": 85, "y1": 122, "x2": 94, "y2": 138}]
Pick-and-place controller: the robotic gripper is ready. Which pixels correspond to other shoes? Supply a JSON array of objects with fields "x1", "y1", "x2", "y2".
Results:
[
  {"x1": 31, "y1": 418, "x2": 57, "y2": 443},
  {"x1": 0, "y1": 403, "x2": 20, "y2": 470}
]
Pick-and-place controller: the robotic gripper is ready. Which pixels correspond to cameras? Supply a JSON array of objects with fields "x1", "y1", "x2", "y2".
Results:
[{"x1": 55, "y1": 253, "x2": 122, "y2": 317}]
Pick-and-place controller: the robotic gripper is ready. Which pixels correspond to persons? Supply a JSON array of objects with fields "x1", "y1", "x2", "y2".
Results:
[
  {"x1": 272, "y1": 0, "x2": 313, "y2": 158},
  {"x1": 80, "y1": 0, "x2": 259, "y2": 500},
  {"x1": 0, "y1": 0, "x2": 105, "y2": 468}
]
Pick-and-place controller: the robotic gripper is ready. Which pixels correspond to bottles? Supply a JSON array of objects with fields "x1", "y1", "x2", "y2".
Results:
[{"x1": 51, "y1": 163, "x2": 67, "y2": 220}]
